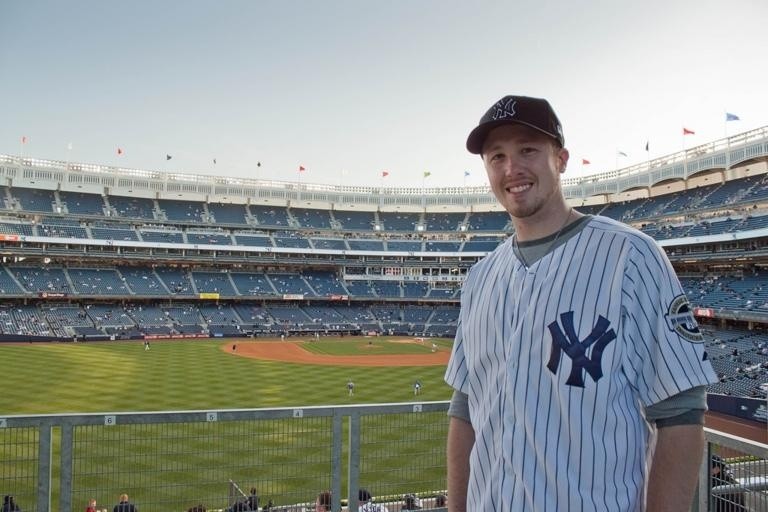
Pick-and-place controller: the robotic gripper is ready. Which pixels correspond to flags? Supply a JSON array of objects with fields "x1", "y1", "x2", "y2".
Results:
[
  {"x1": 725, "y1": 112, "x2": 740, "y2": 121},
  {"x1": 68, "y1": 143, "x2": 73, "y2": 150},
  {"x1": 300, "y1": 166, "x2": 306, "y2": 170},
  {"x1": 166, "y1": 154, "x2": 173, "y2": 161},
  {"x1": 644, "y1": 140, "x2": 648, "y2": 151},
  {"x1": 257, "y1": 162, "x2": 261, "y2": 167},
  {"x1": 424, "y1": 171, "x2": 431, "y2": 177},
  {"x1": 383, "y1": 171, "x2": 389, "y2": 176},
  {"x1": 213, "y1": 158, "x2": 217, "y2": 165},
  {"x1": 21, "y1": 137, "x2": 27, "y2": 144},
  {"x1": 117, "y1": 148, "x2": 122, "y2": 155},
  {"x1": 683, "y1": 128, "x2": 695, "y2": 135},
  {"x1": 464, "y1": 169, "x2": 470, "y2": 177},
  {"x1": 582, "y1": 159, "x2": 590, "y2": 164},
  {"x1": 615, "y1": 148, "x2": 627, "y2": 157}
]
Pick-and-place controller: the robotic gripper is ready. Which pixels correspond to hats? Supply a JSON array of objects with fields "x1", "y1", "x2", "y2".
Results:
[{"x1": 466, "y1": 95, "x2": 563, "y2": 154}]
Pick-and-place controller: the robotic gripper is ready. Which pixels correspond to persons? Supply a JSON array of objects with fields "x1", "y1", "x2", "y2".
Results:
[
  {"x1": 3, "y1": 494, "x2": 20, "y2": 511},
  {"x1": 431, "y1": 341, "x2": 438, "y2": 355},
  {"x1": 143, "y1": 339, "x2": 150, "y2": 351},
  {"x1": 712, "y1": 453, "x2": 745, "y2": 512},
  {"x1": 230, "y1": 343, "x2": 238, "y2": 355},
  {"x1": 441, "y1": 96, "x2": 720, "y2": 511},
  {"x1": 413, "y1": 378, "x2": 421, "y2": 395},
  {"x1": 2, "y1": 184, "x2": 510, "y2": 263},
  {"x1": 345, "y1": 379, "x2": 356, "y2": 396},
  {"x1": 0, "y1": 255, "x2": 472, "y2": 339},
  {"x1": 402, "y1": 491, "x2": 450, "y2": 511},
  {"x1": 229, "y1": 488, "x2": 273, "y2": 511},
  {"x1": 315, "y1": 488, "x2": 387, "y2": 512},
  {"x1": 662, "y1": 236, "x2": 768, "y2": 401},
  {"x1": 87, "y1": 492, "x2": 136, "y2": 511},
  {"x1": 576, "y1": 174, "x2": 767, "y2": 239}
]
